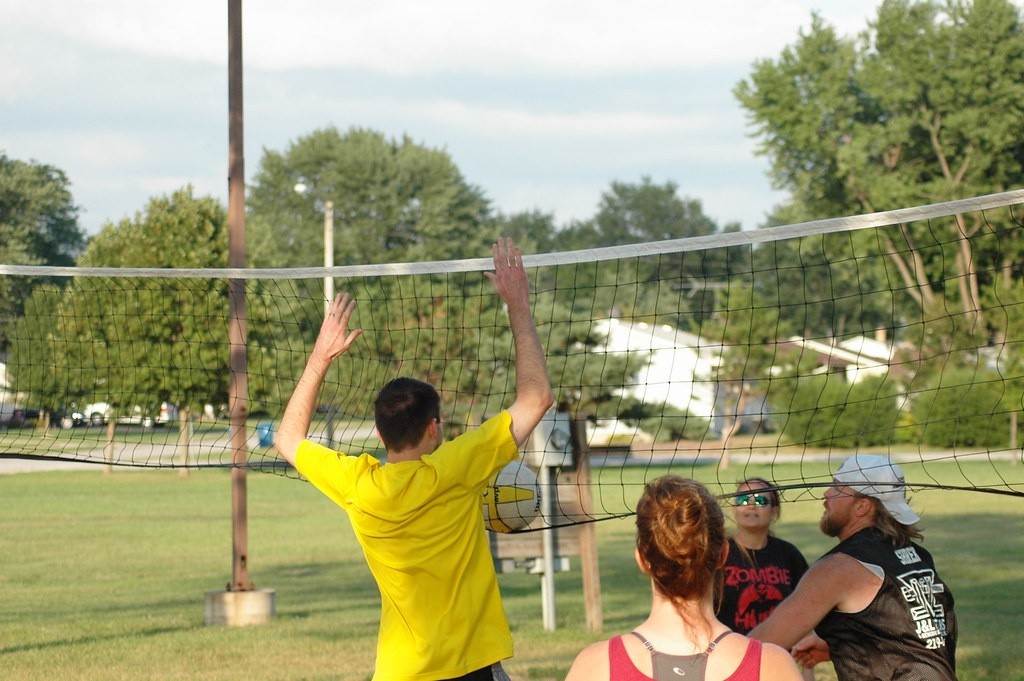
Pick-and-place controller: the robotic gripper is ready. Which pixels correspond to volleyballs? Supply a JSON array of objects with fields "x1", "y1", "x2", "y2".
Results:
[{"x1": 480, "y1": 462, "x2": 541, "y2": 534}]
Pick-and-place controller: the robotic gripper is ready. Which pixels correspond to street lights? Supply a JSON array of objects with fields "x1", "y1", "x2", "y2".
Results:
[{"x1": 293, "y1": 182, "x2": 337, "y2": 451}]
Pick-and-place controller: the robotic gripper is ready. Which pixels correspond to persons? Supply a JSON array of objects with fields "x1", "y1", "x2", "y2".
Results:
[
  {"x1": 745, "y1": 452, "x2": 958, "y2": 681},
  {"x1": 271, "y1": 233, "x2": 556, "y2": 681},
  {"x1": 713, "y1": 478, "x2": 811, "y2": 681},
  {"x1": 561, "y1": 477, "x2": 806, "y2": 681}
]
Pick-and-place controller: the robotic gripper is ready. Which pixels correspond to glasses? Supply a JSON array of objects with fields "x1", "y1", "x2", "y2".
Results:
[{"x1": 734, "y1": 494, "x2": 770, "y2": 507}]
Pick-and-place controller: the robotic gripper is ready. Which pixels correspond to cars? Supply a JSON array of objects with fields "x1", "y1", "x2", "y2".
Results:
[{"x1": 12, "y1": 399, "x2": 178, "y2": 429}]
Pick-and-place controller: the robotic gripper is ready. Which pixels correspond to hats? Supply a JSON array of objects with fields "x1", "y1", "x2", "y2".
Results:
[{"x1": 833, "y1": 453, "x2": 921, "y2": 525}]
